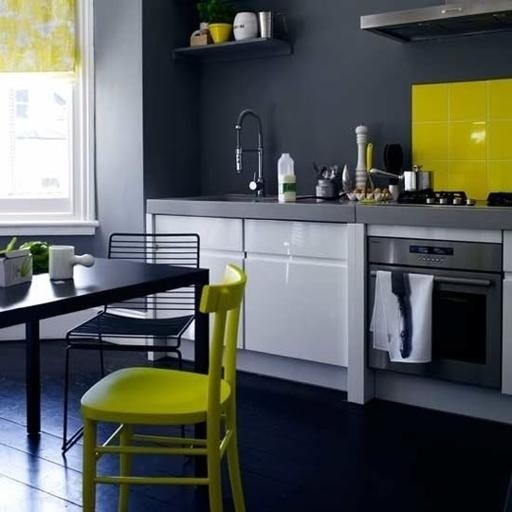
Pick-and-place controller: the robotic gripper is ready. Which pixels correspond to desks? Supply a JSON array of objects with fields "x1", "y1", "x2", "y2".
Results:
[{"x1": 0, "y1": 257, "x2": 209, "y2": 492}]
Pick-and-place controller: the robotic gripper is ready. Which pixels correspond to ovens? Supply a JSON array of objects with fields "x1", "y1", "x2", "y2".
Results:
[{"x1": 367, "y1": 237, "x2": 502, "y2": 392}]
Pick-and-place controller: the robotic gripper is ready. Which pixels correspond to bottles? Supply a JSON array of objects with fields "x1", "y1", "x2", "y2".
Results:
[
  {"x1": 315, "y1": 178, "x2": 335, "y2": 199},
  {"x1": 277, "y1": 153, "x2": 297, "y2": 202},
  {"x1": 233, "y1": 11, "x2": 259, "y2": 41}
]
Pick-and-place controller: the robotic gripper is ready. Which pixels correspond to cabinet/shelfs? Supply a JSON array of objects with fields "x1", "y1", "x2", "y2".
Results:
[
  {"x1": 501, "y1": 231, "x2": 512, "y2": 425},
  {"x1": 154, "y1": 214, "x2": 348, "y2": 392}
]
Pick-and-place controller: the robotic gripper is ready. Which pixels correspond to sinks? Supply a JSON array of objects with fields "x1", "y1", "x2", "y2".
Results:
[{"x1": 197, "y1": 193, "x2": 275, "y2": 202}]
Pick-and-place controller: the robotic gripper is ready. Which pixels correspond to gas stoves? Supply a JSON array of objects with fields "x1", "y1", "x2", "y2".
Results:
[{"x1": 359, "y1": 190, "x2": 512, "y2": 210}]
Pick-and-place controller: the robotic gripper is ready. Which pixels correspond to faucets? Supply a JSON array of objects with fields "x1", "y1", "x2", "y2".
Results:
[{"x1": 234, "y1": 110, "x2": 265, "y2": 196}]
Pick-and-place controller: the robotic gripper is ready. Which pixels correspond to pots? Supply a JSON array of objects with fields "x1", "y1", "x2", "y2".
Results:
[{"x1": 369, "y1": 161, "x2": 434, "y2": 194}]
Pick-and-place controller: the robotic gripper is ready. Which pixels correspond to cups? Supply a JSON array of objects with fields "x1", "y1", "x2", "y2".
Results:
[
  {"x1": 208, "y1": 23, "x2": 232, "y2": 45},
  {"x1": 258, "y1": 11, "x2": 274, "y2": 39},
  {"x1": 49, "y1": 245, "x2": 94, "y2": 281}
]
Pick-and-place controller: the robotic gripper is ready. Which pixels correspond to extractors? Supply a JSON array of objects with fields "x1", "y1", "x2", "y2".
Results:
[{"x1": 360, "y1": 0, "x2": 512, "y2": 47}]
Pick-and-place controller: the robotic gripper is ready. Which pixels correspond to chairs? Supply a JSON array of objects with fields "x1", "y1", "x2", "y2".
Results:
[
  {"x1": 78, "y1": 262, "x2": 246, "y2": 511},
  {"x1": 62, "y1": 232, "x2": 203, "y2": 453}
]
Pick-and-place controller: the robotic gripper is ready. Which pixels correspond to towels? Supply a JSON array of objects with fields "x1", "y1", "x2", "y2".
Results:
[{"x1": 369, "y1": 270, "x2": 435, "y2": 364}]
